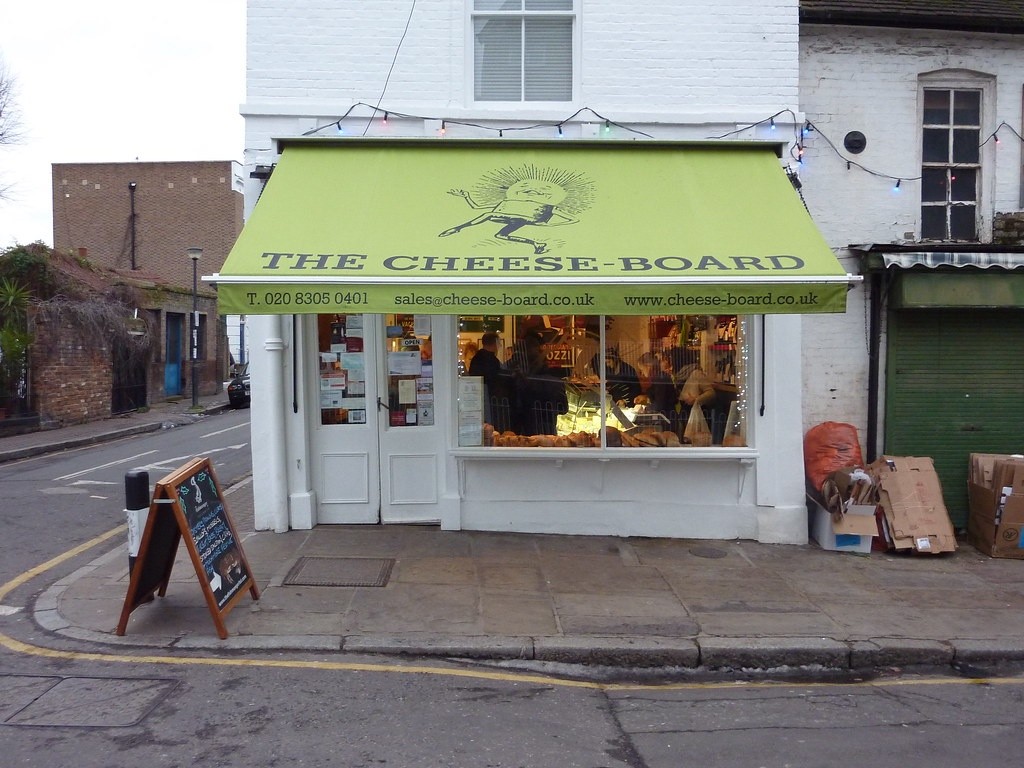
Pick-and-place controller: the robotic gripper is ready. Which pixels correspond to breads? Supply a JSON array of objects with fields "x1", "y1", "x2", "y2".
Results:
[
  {"x1": 723, "y1": 434, "x2": 746, "y2": 446},
  {"x1": 618, "y1": 430, "x2": 680, "y2": 447},
  {"x1": 492, "y1": 429, "x2": 601, "y2": 447},
  {"x1": 690, "y1": 431, "x2": 712, "y2": 447}
]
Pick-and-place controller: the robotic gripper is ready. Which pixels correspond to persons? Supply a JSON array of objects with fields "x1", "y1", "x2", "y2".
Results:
[
  {"x1": 634, "y1": 352, "x2": 679, "y2": 433},
  {"x1": 605, "y1": 348, "x2": 642, "y2": 408},
  {"x1": 468, "y1": 333, "x2": 502, "y2": 424},
  {"x1": 490, "y1": 327, "x2": 569, "y2": 436},
  {"x1": 669, "y1": 347, "x2": 716, "y2": 444}
]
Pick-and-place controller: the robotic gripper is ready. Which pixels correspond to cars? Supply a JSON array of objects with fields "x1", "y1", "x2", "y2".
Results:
[{"x1": 228, "y1": 362, "x2": 251, "y2": 409}]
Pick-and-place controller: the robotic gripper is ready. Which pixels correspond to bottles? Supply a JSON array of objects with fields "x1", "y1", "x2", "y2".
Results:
[
  {"x1": 715, "y1": 354, "x2": 722, "y2": 383},
  {"x1": 723, "y1": 348, "x2": 733, "y2": 382},
  {"x1": 718, "y1": 318, "x2": 737, "y2": 343},
  {"x1": 688, "y1": 324, "x2": 694, "y2": 346},
  {"x1": 730, "y1": 357, "x2": 735, "y2": 386}
]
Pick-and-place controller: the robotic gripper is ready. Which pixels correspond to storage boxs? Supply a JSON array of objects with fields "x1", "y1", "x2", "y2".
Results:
[{"x1": 807, "y1": 493, "x2": 879, "y2": 555}]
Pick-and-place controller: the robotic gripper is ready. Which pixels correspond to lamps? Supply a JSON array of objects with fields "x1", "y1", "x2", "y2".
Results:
[{"x1": 129, "y1": 182, "x2": 137, "y2": 190}]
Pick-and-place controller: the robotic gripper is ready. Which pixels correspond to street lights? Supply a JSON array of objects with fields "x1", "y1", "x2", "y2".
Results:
[{"x1": 186, "y1": 247, "x2": 204, "y2": 408}]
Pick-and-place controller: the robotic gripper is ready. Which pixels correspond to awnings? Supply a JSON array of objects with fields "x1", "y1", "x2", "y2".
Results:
[
  {"x1": 848, "y1": 243, "x2": 1024, "y2": 270},
  {"x1": 200, "y1": 150, "x2": 864, "y2": 316}
]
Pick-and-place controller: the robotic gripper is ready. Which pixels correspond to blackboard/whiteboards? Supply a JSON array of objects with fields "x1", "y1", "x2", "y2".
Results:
[{"x1": 128, "y1": 457, "x2": 254, "y2": 623}]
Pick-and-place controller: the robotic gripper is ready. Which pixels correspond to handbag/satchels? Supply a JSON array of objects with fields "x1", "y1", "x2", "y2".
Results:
[{"x1": 683, "y1": 398, "x2": 711, "y2": 444}]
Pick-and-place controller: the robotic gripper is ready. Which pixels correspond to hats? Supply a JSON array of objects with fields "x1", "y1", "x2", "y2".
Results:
[
  {"x1": 605, "y1": 348, "x2": 617, "y2": 360},
  {"x1": 482, "y1": 332, "x2": 503, "y2": 347}
]
